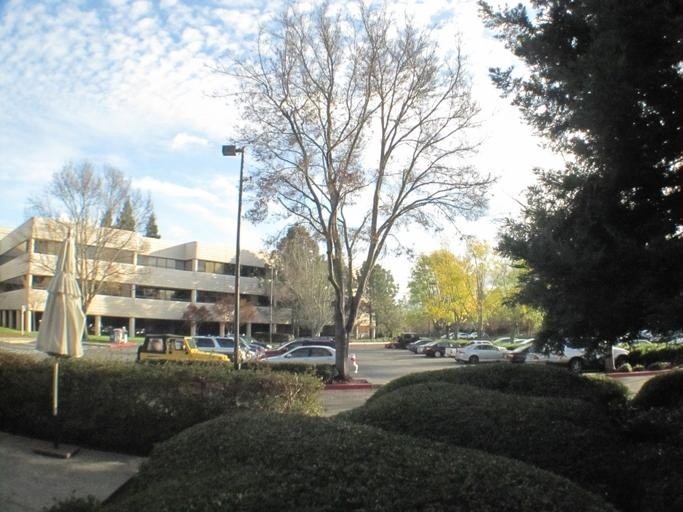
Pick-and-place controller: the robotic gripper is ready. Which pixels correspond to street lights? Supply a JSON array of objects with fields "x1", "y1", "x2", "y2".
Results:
[
  {"x1": 218, "y1": 142, "x2": 245, "y2": 372},
  {"x1": 265, "y1": 256, "x2": 286, "y2": 346}
]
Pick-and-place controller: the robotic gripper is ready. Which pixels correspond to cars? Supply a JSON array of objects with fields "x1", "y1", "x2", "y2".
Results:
[
  {"x1": 190, "y1": 333, "x2": 361, "y2": 383},
  {"x1": 386, "y1": 325, "x2": 682, "y2": 376}
]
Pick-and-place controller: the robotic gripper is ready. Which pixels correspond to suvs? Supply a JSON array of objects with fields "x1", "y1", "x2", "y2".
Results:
[{"x1": 135, "y1": 331, "x2": 232, "y2": 368}]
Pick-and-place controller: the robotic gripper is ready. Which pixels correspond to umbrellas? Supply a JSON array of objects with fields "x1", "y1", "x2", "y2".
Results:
[{"x1": 37, "y1": 230, "x2": 84, "y2": 414}]
